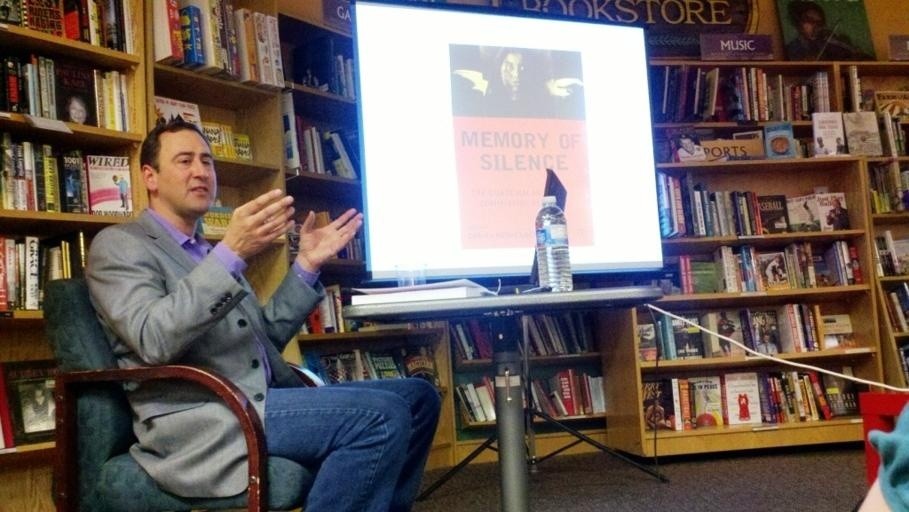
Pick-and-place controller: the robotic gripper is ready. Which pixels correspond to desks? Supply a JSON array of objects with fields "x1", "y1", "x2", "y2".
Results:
[{"x1": 343, "y1": 285, "x2": 665, "y2": 512}]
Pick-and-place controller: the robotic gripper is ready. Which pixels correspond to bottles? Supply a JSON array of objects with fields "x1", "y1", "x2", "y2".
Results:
[{"x1": 536, "y1": 194, "x2": 574, "y2": 293}]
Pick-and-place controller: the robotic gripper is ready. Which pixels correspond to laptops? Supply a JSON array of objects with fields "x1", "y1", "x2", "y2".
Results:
[{"x1": 485, "y1": 169, "x2": 566, "y2": 294}]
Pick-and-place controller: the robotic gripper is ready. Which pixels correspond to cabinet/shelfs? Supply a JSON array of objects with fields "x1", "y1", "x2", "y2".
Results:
[{"x1": 1, "y1": 1, "x2": 909, "y2": 460}]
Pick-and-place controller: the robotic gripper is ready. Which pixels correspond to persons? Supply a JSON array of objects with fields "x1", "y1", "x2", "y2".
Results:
[
  {"x1": 855, "y1": 403, "x2": 909, "y2": 512},
  {"x1": 455, "y1": 50, "x2": 582, "y2": 118},
  {"x1": 783, "y1": 0, "x2": 855, "y2": 61},
  {"x1": 86, "y1": 111, "x2": 441, "y2": 511}
]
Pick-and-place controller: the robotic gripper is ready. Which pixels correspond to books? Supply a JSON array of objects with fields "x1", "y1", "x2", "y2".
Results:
[
  {"x1": 284, "y1": 55, "x2": 364, "y2": 260},
  {"x1": 638, "y1": 64, "x2": 908, "y2": 432},
  {"x1": 155, "y1": 0, "x2": 285, "y2": 164},
  {"x1": 1, "y1": 0, "x2": 133, "y2": 312},
  {"x1": 1, "y1": 362, "x2": 60, "y2": 447},
  {"x1": 301, "y1": 280, "x2": 497, "y2": 386},
  {"x1": 447, "y1": 311, "x2": 607, "y2": 425},
  {"x1": 203, "y1": 207, "x2": 234, "y2": 237}
]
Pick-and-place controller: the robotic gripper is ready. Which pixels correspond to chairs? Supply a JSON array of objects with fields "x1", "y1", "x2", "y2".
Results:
[{"x1": 43, "y1": 278, "x2": 313, "y2": 512}]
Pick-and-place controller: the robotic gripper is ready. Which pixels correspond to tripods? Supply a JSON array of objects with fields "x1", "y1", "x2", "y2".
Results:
[{"x1": 415, "y1": 312, "x2": 666, "y2": 502}]
